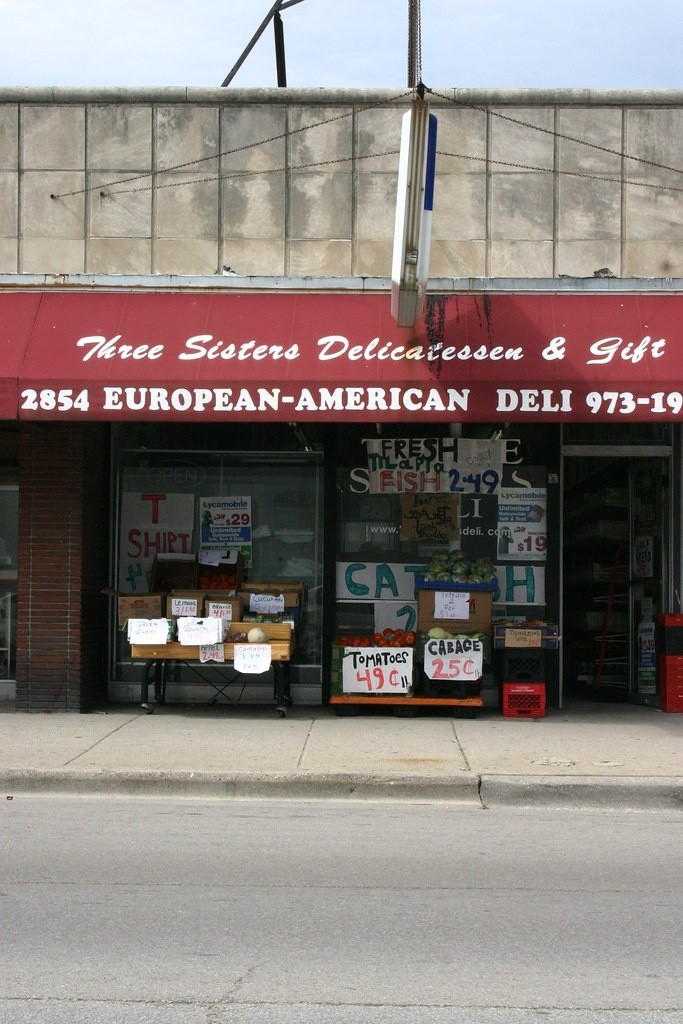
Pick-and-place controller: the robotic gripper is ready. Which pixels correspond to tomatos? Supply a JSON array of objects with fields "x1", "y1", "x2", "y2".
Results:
[{"x1": 337, "y1": 627, "x2": 416, "y2": 647}]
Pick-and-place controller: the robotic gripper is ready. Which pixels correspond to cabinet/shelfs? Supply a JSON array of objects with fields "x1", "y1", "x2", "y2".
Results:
[{"x1": 564, "y1": 487, "x2": 639, "y2": 698}]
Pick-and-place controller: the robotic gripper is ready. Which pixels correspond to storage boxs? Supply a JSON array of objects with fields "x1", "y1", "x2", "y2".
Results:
[
  {"x1": 117, "y1": 549, "x2": 306, "y2": 662},
  {"x1": 330, "y1": 574, "x2": 558, "y2": 718},
  {"x1": 655, "y1": 612, "x2": 683, "y2": 713}
]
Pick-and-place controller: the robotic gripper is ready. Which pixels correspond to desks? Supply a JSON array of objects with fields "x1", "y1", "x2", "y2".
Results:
[{"x1": 139, "y1": 658, "x2": 294, "y2": 718}]
